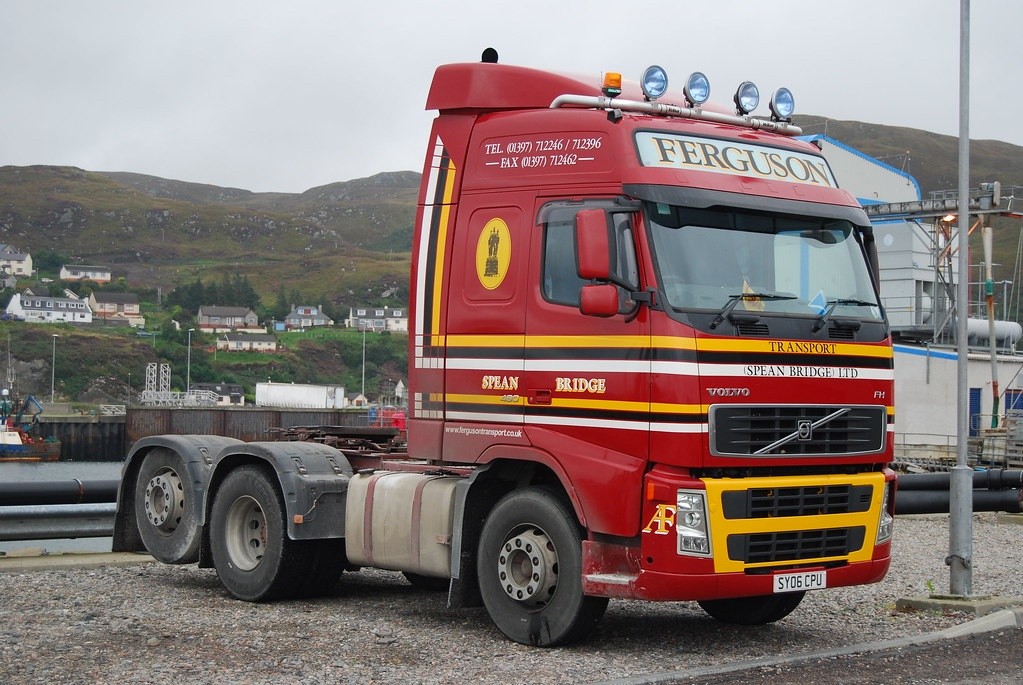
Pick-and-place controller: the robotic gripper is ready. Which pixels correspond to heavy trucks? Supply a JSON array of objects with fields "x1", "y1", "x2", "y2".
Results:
[{"x1": 108, "y1": 49, "x2": 897, "y2": 647}]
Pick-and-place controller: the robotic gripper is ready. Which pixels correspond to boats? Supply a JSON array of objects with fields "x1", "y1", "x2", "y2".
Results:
[{"x1": 0, "y1": 333, "x2": 62, "y2": 462}]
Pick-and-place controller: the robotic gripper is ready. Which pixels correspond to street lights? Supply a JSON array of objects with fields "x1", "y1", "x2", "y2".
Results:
[
  {"x1": 360, "y1": 320, "x2": 370, "y2": 407},
  {"x1": 51, "y1": 334, "x2": 58, "y2": 404},
  {"x1": 186, "y1": 328, "x2": 196, "y2": 392}
]
